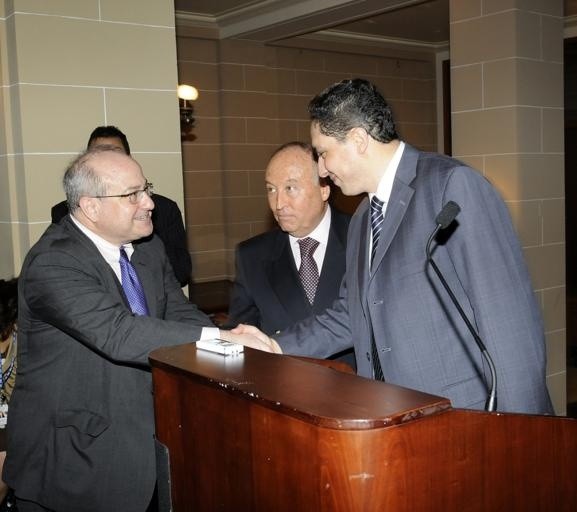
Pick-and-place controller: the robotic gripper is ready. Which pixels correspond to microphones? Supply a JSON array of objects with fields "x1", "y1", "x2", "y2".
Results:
[{"x1": 425, "y1": 200, "x2": 497, "y2": 413}]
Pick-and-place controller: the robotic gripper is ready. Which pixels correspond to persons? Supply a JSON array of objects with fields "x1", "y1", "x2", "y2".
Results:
[
  {"x1": 0, "y1": 274, "x2": 18, "y2": 508},
  {"x1": 1, "y1": 144, "x2": 276, "y2": 509},
  {"x1": 216, "y1": 140, "x2": 360, "y2": 373},
  {"x1": 228, "y1": 77, "x2": 558, "y2": 417},
  {"x1": 51, "y1": 124, "x2": 194, "y2": 289}
]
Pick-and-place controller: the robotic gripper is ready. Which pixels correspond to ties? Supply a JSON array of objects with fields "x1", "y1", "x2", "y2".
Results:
[
  {"x1": 368, "y1": 195, "x2": 385, "y2": 274},
  {"x1": 118, "y1": 247, "x2": 150, "y2": 318},
  {"x1": 296, "y1": 237, "x2": 321, "y2": 308}
]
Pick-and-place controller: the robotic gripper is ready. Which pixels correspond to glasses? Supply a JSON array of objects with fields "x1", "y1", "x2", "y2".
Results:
[{"x1": 82, "y1": 182, "x2": 154, "y2": 204}]
